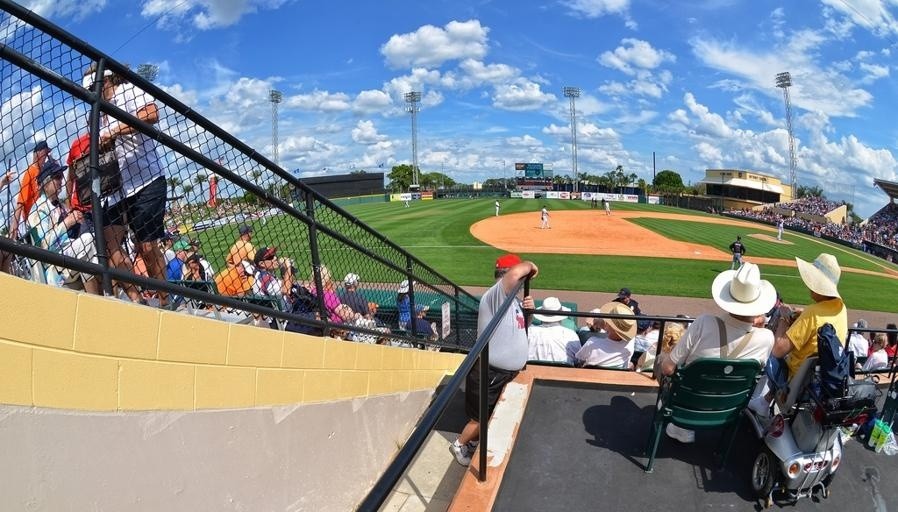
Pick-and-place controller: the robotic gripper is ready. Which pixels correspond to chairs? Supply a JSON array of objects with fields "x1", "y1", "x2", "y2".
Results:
[{"x1": 643, "y1": 357, "x2": 762, "y2": 474}]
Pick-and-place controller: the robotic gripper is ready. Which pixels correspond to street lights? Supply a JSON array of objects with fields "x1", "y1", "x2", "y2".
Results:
[
  {"x1": 137, "y1": 64, "x2": 157, "y2": 81},
  {"x1": 404, "y1": 92, "x2": 420, "y2": 186},
  {"x1": 564, "y1": 87, "x2": 580, "y2": 193},
  {"x1": 777, "y1": 72, "x2": 798, "y2": 200},
  {"x1": 268, "y1": 90, "x2": 285, "y2": 202}
]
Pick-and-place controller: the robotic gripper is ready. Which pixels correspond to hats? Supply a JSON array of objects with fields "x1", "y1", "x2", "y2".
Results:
[
  {"x1": 796, "y1": 253, "x2": 843, "y2": 301},
  {"x1": 858, "y1": 319, "x2": 867, "y2": 328},
  {"x1": 160, "y1": 230, "x2": 203, "y2": 264},
  {"x1": 36, "y1": 160, "x2": 67, "y2": 183},
  {"x1": 369, "y1": 303, "x2": 379, "y2": 310},
  {"x1": 711, "y1": 261, "x2": 777, "y2": 317},
  {"x1": 496, "y1": 255, "x2": 520, "y2": 267},
  {"x1": 415, "y1": 304, "x2": 429, "y2": 314},
  {"x1": 239, "y1": 226, "x2": 253, "y2": 234},
  {"x1": 345, "y1": 273, "x2": 359, "y2": 286},
  {"x1": 616, "y1": 288, "x2": 631, "y2": 298},
  {"x1": 585, "y1": 301, "x2": 655, "y2": 342},
  {"x1": 398, "y1": 280, "x2": 416, "y2": 294},
  {"x1": 83, "y1": 69, "x2": 112, "y2": 90},
  {"x1": 34, "y1": 141, "x2": 52, "y2": 152},
  {"x1": 242, "y1": 247, "x2": 298, "y2": 274},
  {"x1": 533, "y1": 297, "x2": 572, "y2": 322}
]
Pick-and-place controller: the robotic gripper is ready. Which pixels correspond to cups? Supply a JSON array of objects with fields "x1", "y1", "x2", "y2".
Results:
[{"x1": 868, "y1": 420, "x2": 892, "y2": 453}]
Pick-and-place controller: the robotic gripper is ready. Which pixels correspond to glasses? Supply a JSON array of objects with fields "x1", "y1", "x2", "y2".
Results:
[{"x1": 42, "y1": 173, "x2": 61, "y2": 187}]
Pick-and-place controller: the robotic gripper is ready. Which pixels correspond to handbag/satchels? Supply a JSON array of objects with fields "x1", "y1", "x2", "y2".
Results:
[
  {"x1": 814, "y1": 380, "x2": 877, "y2": 411},
  {"x1": 73, "y1": 135, "x2": 123, "y2": 206},
  {"x1": 817, "y1": 323, "x2": 850, "y2": 396},
  {"x1": 347, "y1": 317, "x2": 381, "y2": 343},
  {"x1": 286, "y1": 310, "x2": 322, "y2": 336}
]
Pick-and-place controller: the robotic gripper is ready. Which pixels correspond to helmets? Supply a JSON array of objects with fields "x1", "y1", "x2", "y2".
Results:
[{"x1": 737, "y1": 236, "x2": 741, "y2": 240}]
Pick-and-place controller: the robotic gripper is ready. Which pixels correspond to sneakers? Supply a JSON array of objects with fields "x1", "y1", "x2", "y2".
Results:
[{"x1": 450, "y1": 434, "x2": 479, "y2": 466}]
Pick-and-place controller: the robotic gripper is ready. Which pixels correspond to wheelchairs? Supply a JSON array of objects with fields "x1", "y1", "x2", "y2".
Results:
[{"x1": 746, "y1": 350, "x2": 883, "y2": 509}]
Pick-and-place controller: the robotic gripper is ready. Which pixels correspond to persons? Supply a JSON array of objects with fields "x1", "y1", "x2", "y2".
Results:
[
  {"x1": 591, "y1": 198, "x2": 611, "y2": 215},
  {"x1": 848, "y1": 319, "x2": 898, "y2": 372},
  {"x1": 730, "y1": 235, "x2": 745, "y2": 268},
  {"x1": 766, "y1": 291, "x2": 792, "y2": 332},
  {"x1": 450, "y1": 254, "x2": 540, "y2": 465},
  {"x1": 529, "y1": 288, "x2": 690, "y2": 375},
  {"x1": 661, "y1": 263, "x2": 777, "y2": 443},
  {"x1": 0, "y1": 60, "x2": 168, "y2": 309},
  {"x1": 162, "y1": 224, "x2": 436, "y2": 350},
  {"x1": 730, "y1": 195, "x2": 898, "y2": 262},
  {"x1": 542, "y1": 206, "x2": 551, "y2": 228},
  {"x1": 496, "y1": 200, "x2": 501, "y2": 216},
  {"x1": 749, "y1": 253, "x2": 848, "y2": 417}
]
